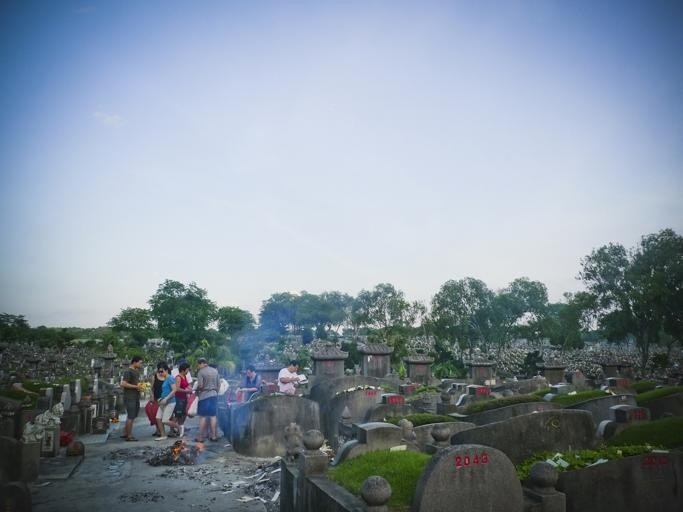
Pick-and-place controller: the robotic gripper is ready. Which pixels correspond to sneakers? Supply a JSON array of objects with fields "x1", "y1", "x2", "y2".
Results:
[
  {"x1": 168, "y1": 427, "x2": 191, "y2": 440},
  {"x1": 196, "y1": 432, "x2": 221, "y2": 443},
  {"x1": 119, "y1": 435, "x2": 138, "y2": 441},
  {"x1": 153, "y1": 432, "x2": 160, "y2": 437},
  {"x1": 155, "y1": 435, "x2": 168, "y2": 442}
]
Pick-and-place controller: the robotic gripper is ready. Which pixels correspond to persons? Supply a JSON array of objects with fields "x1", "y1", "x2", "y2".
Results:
[
  {"x1": 277, "y1": 359, "x2": 301, "y2": 395},
  {"x1": 117, "y1": 354, "x2": 222, "y2": 446},
  {"x1": 234, "y1": 365, "x2": 262, "y2": 403}
]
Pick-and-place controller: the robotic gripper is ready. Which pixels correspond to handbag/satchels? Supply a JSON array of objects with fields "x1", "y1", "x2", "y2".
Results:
[
  {"x1": 217, "y1": 378, "x2": 229, "y2": 395},
  {"x1": 185, "y1": 392, "x2": 199, "y2": 418}
]
